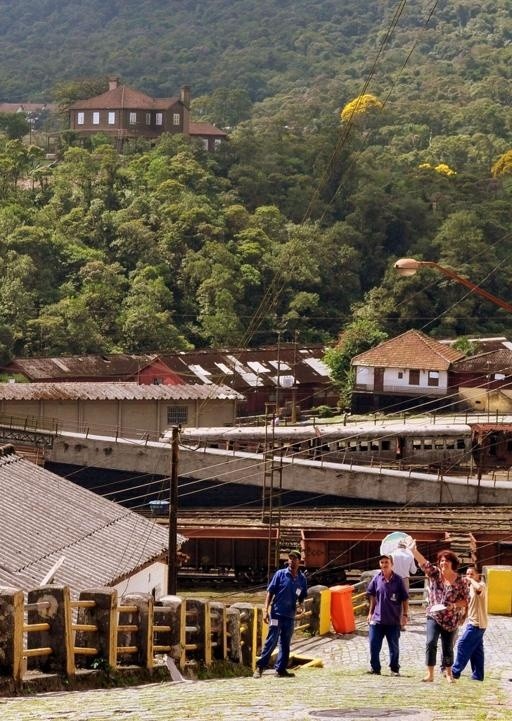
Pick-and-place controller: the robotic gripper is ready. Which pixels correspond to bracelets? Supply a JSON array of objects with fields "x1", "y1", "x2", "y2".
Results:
[
  {"x1": 452, "y1": 604, "x2": 456, "y2": 611},
  {"x1": 403, "y1": 614, "x2": 407, "y2": 617}
]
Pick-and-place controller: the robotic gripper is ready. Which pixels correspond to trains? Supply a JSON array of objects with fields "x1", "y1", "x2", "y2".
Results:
[
  {"x1": 175, "y1": 542, "x2": 512, "y2": 587},
  {"x1": 156, "y1": 423, "x2": 512, "y2": 470}
]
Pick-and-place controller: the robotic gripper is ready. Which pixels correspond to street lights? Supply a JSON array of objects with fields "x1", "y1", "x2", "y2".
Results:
[{"x1": 393, "y1": 256, "x2": 511, "y2": 312}]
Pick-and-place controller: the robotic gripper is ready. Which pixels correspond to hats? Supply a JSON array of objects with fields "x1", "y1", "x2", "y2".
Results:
[
  {"x1": 288, "y1": 549, "x2": 302, "y2": 561},
  {"x1": 376, "y1": 554, "x2": 394, "y2": 564}
]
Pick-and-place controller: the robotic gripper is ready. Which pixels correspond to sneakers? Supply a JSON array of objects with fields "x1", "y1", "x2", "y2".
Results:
[
  {"x1": 364, "y1": 667, "x2": 382, "y2": 675},
  {"x1": 390, "y1": 669, "x2": 400, "y2": 677},
  {"x1": 418, "y1": 677, "x2": 432, "y2": 683},
  {"x1": 273, "y1": 670, "x2": 295, "y2": 678},
  {"x1": 252, "y1": 666, "x2": 264, "y2": 679},
  {"x1": 447, "y1": 675, "x2": 456, "y2": 683}
]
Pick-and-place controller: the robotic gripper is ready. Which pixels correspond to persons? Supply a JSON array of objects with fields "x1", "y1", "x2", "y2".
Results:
[
  {"x1": 363, "y1": 553, "x2": 409, "y2": 678},
  {"x1": 443, "y1": 565, "x2": 489, "y2": 684},
  {"x1": 400, "y1": 537, "x2": 471, "y2": 684},
  {"x1": 252, "y1": 551, "x2": 309, "y2": 679},
  {"x1": 388, "y1": 540, "x2": 417, "y2": 632}
]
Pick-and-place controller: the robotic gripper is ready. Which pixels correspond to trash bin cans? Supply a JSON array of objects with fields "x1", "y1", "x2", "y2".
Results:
[{"x1": 330, "y1": 585, "x2": 356, "y2": 633}]
